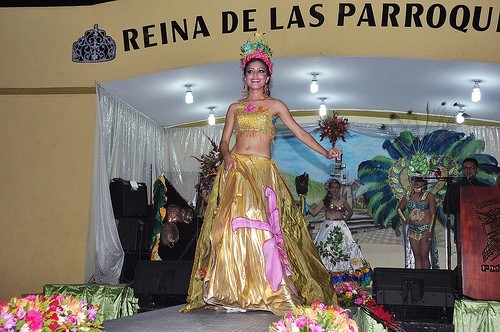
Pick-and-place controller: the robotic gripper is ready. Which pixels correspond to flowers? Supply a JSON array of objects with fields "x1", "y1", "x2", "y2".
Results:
[
  {"x1": 0, "y1": 289, "x2": 107, "y2": 332},
  {"x1": 191, "y1": 132, "x2": 224, "y2": 219},
  {"x1": 332, "y1": 258, "x2": 371, "y2": 306},
  {"x1": 269, "y1": 303, "x2": 359, "y2": 332},
  {"x1": 314, "y1": 109, "x2": 351, "y2": 146}
]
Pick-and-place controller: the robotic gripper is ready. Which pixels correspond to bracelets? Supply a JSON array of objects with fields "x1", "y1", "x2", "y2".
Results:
[{"x1": 223, "y1": 154, "x2": 229, "y2": 159}]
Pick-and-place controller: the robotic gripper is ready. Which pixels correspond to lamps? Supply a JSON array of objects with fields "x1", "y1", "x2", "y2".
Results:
[
  {"x1": 185, "y1": 84, "x2": 194, "y2": 103},
  {"x1": 456, "y1": 103, "x2": 464, "y2": 123},
  {"x1": 318, "y1": 97, "x2": 327, "y2": 116},
  {"x1": 310, "y1": 73, "x2": 319, "y2": 93},
  {"x1": 208, "y1": 107, "x2": 216, "y2": 124},
  {"x1": 472, "y1": 80, "x2": 481, "y2": 102}
]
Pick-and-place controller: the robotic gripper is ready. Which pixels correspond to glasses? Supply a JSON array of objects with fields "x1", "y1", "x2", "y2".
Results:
[{"x1": 462, "y1": 166, "x2": 477, "y2": 170}]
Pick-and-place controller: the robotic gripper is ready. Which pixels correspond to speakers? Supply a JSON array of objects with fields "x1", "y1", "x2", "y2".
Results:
[
  {"x1": 134, "y1": 261, "x2": 192, "y2": 296},
  {"x1": 109, "y1": 181, "x2": 157, "y2": 273},
  {"x1": 370, "y1": 268, "x2": 460, "y2": 322}
]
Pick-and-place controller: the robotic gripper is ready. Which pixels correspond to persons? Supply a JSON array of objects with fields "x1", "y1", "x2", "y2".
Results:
[
  {"x1": 300, "y1": 178, "x2": 373, "y2": 289},
  {"x1": 443, "y1": 158, "x2": 490, "y2": 297},
  {"x1": 396, "y1": 170, "x2": 437, "y2": 270},
  {"x1": 180, "y1": 32, "x2": 343, "y2": 315}
]
